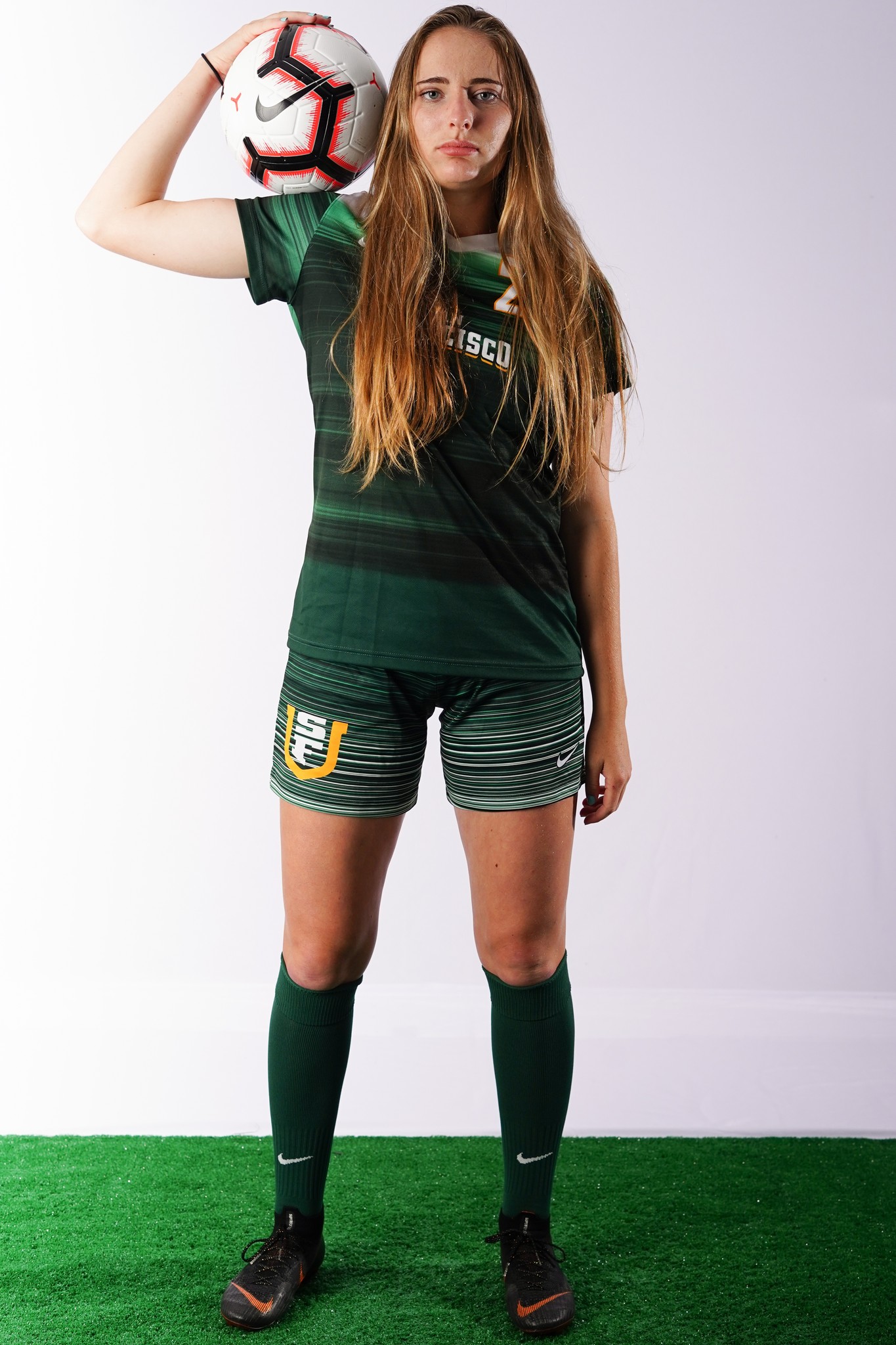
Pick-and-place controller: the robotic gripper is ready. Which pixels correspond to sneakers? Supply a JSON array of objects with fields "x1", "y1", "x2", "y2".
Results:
[
  {"x1": 482, "y1": 1208, "x2": 578, "y2": 1338},
  {"x1": 219, "y1": 1206, "x2": 325, "y2": 1329}
]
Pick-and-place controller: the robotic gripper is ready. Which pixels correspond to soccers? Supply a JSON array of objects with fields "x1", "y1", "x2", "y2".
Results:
[{"x1": 220, "y1": 22, "x2": 389, "y2": 195}]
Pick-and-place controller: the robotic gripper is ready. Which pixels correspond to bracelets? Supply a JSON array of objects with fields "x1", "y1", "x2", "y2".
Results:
[{"x1": 200, "y1": 53, "x2": 225, "y2": 88}]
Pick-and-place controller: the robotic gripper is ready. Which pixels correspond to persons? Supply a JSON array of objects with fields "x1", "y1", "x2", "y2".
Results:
[{"x1": 75, "y1": 3, "x2": 631, "y2": 1332}]
